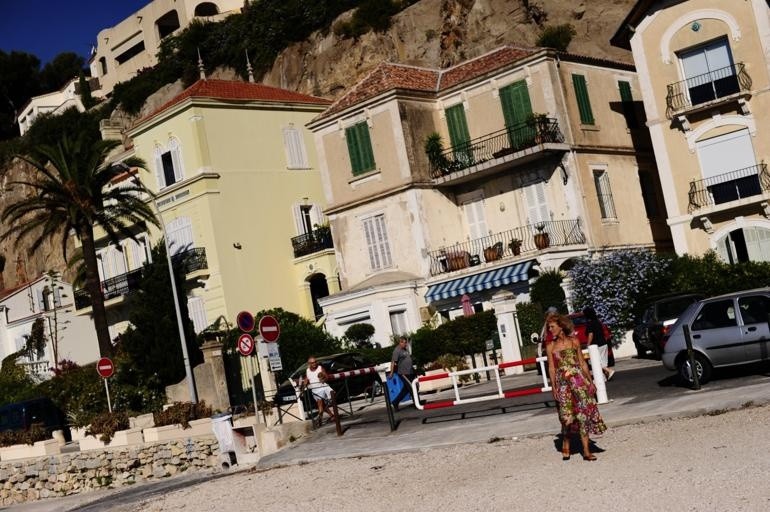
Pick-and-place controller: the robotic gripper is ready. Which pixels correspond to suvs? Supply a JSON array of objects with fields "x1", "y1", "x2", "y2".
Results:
[
  {"x1": 632, "y1": 289, "x2": 718, "y2": 362},
  {"x1": 530, "y1": 303, "x2": 619, "y2": 377}
]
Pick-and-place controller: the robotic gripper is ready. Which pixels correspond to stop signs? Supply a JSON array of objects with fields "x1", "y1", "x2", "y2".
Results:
[
  {"x1": 96, "y1": 357, "x2": 115, "y2": 379},
  {"x1": 258, "y1": 314, "x2": 282, "y2": 343}
]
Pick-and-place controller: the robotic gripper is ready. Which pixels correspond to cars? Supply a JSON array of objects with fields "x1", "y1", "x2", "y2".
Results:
[
  {"x1": 272, "y1": 350, "x2": 385, "y2": 409},
  {"x1": 658, "y1": 286, "x2": 769, "y2": 386}
]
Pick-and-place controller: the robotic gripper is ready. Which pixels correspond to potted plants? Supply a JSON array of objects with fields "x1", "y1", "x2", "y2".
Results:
[
  {"x1": 424, "y1": 135, "x2": 449, "y2": 176},
  {"x1": 534, "y1": 224, "x2": 554, "y2": 248},
  {"x1": 508, "y1": 239, "x2": 522, "y2": 253},
  {"x1": 523, "y1": 113, "x2": 559, "y2": 142}
]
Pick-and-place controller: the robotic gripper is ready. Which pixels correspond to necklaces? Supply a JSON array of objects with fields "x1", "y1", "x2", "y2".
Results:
[{"x1": 555, "y1": 335, "x2": 565, "y2": 345}]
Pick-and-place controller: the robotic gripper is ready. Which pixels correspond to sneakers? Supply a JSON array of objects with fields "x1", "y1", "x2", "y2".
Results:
[{"x1": 608, "y1": 368, "x2": 614, "y2": 380}]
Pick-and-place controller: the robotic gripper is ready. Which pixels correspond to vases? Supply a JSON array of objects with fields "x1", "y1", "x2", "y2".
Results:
[{"x1": 483, "y1": 246, "x2": 500, "y2": 263}]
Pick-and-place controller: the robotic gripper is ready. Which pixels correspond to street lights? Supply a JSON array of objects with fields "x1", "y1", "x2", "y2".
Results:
[{"x1": 109, "y1": 161, "x2": 200, "y2": 404}]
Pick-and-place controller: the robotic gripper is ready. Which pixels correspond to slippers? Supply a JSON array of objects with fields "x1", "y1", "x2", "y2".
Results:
[
  {"x1": 562, "y1": 440, "x2": 570, "y2": 459},
  {"x1": 583, "y1": 455, "x2": 597, "y2": 460}
]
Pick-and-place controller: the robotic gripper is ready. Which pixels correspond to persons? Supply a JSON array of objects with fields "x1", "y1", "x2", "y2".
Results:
[
  {"x1": 583, "y1": 306, "x2": 617, "y2": 385},
  {"x1": 302, "y1": 357, "x2": 336, "y2": 427},
  {"x1": 545, "y1": 312, "x2": 608, "y2": 461},
  {"x1": 388, "y1": 336, "x2": 427, "y2": 412}
]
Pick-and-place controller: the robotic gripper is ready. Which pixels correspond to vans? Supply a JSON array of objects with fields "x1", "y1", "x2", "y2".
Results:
[{"x1": 0, "y1": 396, "x2": 73, "y2": 442}]
